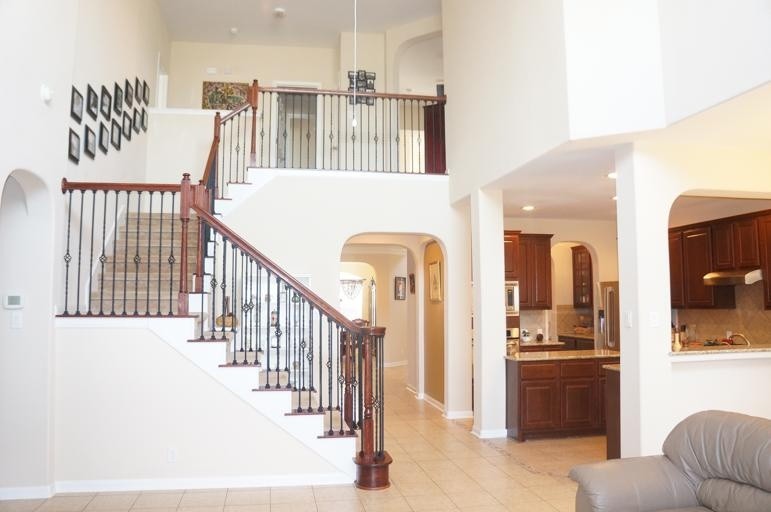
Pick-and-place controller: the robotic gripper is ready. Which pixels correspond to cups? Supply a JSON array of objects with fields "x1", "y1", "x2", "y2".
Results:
[{"x1": 685, "y1": 324, "x2": 696, "y2": 343}]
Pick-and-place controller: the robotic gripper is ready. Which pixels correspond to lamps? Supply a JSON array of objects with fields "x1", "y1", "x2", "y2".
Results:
[{"x1": 352, "y1": 1, "x2": 357, "y2": 126}]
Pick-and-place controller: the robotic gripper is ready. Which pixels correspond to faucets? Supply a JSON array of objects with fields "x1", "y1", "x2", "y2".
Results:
[
  {"x1": 719, "y1": 341, "x2": 730, "y2": 346},
  {"x1": 729, "y1": 333, "x2": 751, "y2": 346}
]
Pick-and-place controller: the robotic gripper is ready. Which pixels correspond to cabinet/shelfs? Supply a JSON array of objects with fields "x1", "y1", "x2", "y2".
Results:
[
  {"x1": 570, "y1": 245, "x2": 593, "y2": 309},
  {"x1": 504, "y1": 229, "x2": 521, "y2": 281},
  {"x1": 505, "y1": 359, "x2": 561, "y2": 442},
  {"x1": 597, "y1": 357, "x2": 620, "y2": 434},
  {"x1": 558, "y1": 337, "x2": 593, "y2": 351},
  {"x1": 561, "y1": 358, "x2": 597, "y2": 435},
  {"x1": 682, "y1": 223, "x2": 736, "y2": 310},
  {"x1": 714, "y1": 212, "x2": 759, "y2": 271},
  {"x1": 519, "y1": 233, "x2": 556, "y2": 310},
  {"x1": 668, "y1": 227, "x2": 684, "y2": 309},
  {"x1": 67, "y1": 76, "x2": 151, "y2": 164},
  {"x1": 760, "y1": 208, "x2": 771, "y2": 310},
  {"x1": 521, "y1": 345, "x2": 565, "y2": 352}
]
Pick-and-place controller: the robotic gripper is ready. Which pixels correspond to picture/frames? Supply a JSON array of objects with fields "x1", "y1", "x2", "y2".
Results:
[
  {"x1": 348, "y1": 71, "x2": 376, "y2": 104},
  {"x1": 395, "y1": 277, "x2": 407, "y2": 300},
  {"x1": 429, "y1": 260, "x2": 442, "y2": 302}
]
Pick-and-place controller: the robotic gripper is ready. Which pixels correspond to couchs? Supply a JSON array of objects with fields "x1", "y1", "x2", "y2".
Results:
[{"x1": 568, "y1": 410, "x2": 771, "y2": 512}]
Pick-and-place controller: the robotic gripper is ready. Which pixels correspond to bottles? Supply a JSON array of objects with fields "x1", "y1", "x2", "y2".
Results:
[{"x1": 673, "y1": 333, "x2": 681, "y2": 352}]
